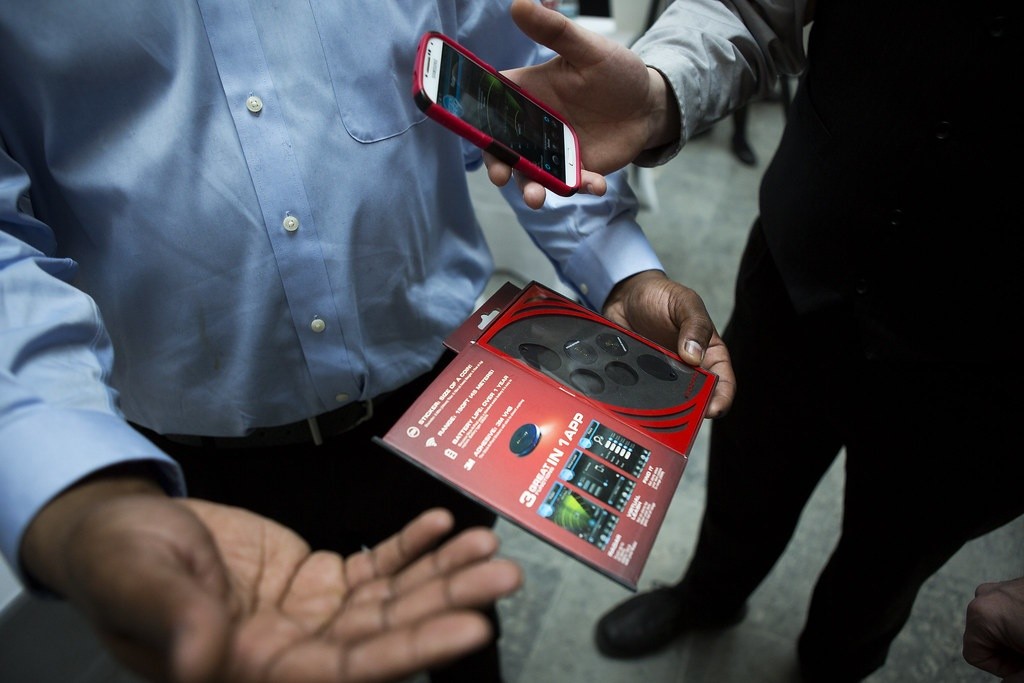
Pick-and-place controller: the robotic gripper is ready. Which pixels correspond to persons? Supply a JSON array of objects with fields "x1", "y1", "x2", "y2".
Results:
[
  {"x1": 0, "y1": 0, "x2": 733, "y2": 683},
  {"x1": 478, "y1": 0, "x2": 1023, "y2": 683}
]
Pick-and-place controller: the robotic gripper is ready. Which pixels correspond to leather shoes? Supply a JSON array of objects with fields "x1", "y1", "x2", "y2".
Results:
[{"x1": 596, "y1": 588, "x2": 739, "y2": 660}]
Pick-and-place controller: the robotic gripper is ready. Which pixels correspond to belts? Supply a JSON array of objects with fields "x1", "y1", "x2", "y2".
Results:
[{"x1": 128, "y1": 351, "x2": 452, "y2": 461}]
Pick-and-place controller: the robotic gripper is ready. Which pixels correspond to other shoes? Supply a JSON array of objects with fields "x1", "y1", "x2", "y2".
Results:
[{"x1": 729, "y1": 134, "x2": 757, "y2": 165}]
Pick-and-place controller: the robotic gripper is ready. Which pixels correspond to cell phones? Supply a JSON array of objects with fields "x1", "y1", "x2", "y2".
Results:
[{"x1": 414, "y1": 31, "x2": 582, "y2": 196}]
